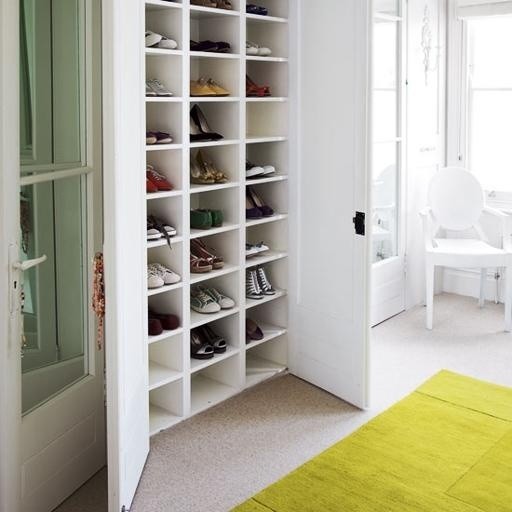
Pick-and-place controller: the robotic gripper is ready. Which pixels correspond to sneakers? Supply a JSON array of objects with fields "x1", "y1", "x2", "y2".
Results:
[
  {"x1": 207, "y1": 78, "x2": 230, "y2": 96},
  {"x1": 148, "y1": 263, "x2": 181, "y2": 288},
  {"x1": 146, "y1": 79, "x2": 174, "y2": 96},
  {"x1": 246, "y1": 159, "x2": 276, "y2": 178},
  {"x1": 190, "y1": 77, "x2": 217, "y2": 96},
  {"x1": 191, "y1": 284, "x2": 235, "y2": 313},
  {"x1": 147, "y1": 216, "x2": 177, "y2": 250}
]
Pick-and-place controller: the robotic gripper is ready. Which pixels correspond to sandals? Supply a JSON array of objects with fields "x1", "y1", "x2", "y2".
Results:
[
  {"x1": 246, "y1": 270, "x2": 264, "y2": 299},
  {"x1": 257, "y1": 267, "x2": 276, "y2": 295}
]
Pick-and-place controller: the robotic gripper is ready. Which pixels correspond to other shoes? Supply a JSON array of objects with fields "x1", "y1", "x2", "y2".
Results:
[
  {"x1": 194, "y1": 1, "x2": 232, "y2": 10},
  {"x1": 146, "y1": 132, "x2": 173, "y2": 144},
  {"x1": 190, "y1": 39, "x2": 231, "y2": 52},
  {"x1": 246, "y1": 39, "x2": 272, "y2": 56},
  {"x1": 146, "y1": 161, "x2": 174, "y2": 191},
  {"x1": 246, "y1": 75, "x2": 272, "y2": 96},
  {"x1": 246, "y1": 317, "x2": 264, "y2": 343},
  {"x1": 153, "y1": 35, "x2": 177, "y2": 49},
  {"x1": 148, "y1": 311, "x2": 180, "y2": 336},
  {"x1": 191, "y1": 209, "x2": 223, "y2": 230},
  {"x1": 246, "y1": 240, "x2": 270, "y2": 258},
  {"x1": 247, "y1": 5, "x2": 268, "y2": 15},
  {"x1": 146, "y1": 31, "x2": 162, "y2": 47}
]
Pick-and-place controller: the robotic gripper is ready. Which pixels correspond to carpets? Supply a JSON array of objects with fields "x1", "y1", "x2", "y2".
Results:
[{"x1": 229, "y1": 370, "x2": 512, "y2": 512}]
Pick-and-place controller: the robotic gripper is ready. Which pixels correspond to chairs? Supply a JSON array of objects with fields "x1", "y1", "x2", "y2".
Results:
[{"x1": 419, "y1": 168, "x2": 512, "y2": 331}]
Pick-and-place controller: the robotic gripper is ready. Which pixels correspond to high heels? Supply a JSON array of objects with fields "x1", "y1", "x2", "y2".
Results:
[
  {"x1": 197, "y1": 147, "x2": 230, "y2": 183},
  {"x1": 190, "y1": 239, "x2": 224, "y2": 273},
  {"x1": 190, "y1": 103, "x2": 224, "y2": 140},
  {"x1": 246, "y1": 187, "x2": 273, "y2": 219},
  {"x1": 191, "y1": 325, "x2": 226, "y2": 359},
  {"x1": 190, "y1": 160, "x2": 215, "y2": 185},
  {"x1": 190, "y1": 116, "x2": 205, "y2": 140}
]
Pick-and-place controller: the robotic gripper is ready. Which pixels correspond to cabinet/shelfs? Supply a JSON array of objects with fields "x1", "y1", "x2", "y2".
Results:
[{"x1": 101, "y1": 0, "x2": 373, "y2": 512}]
